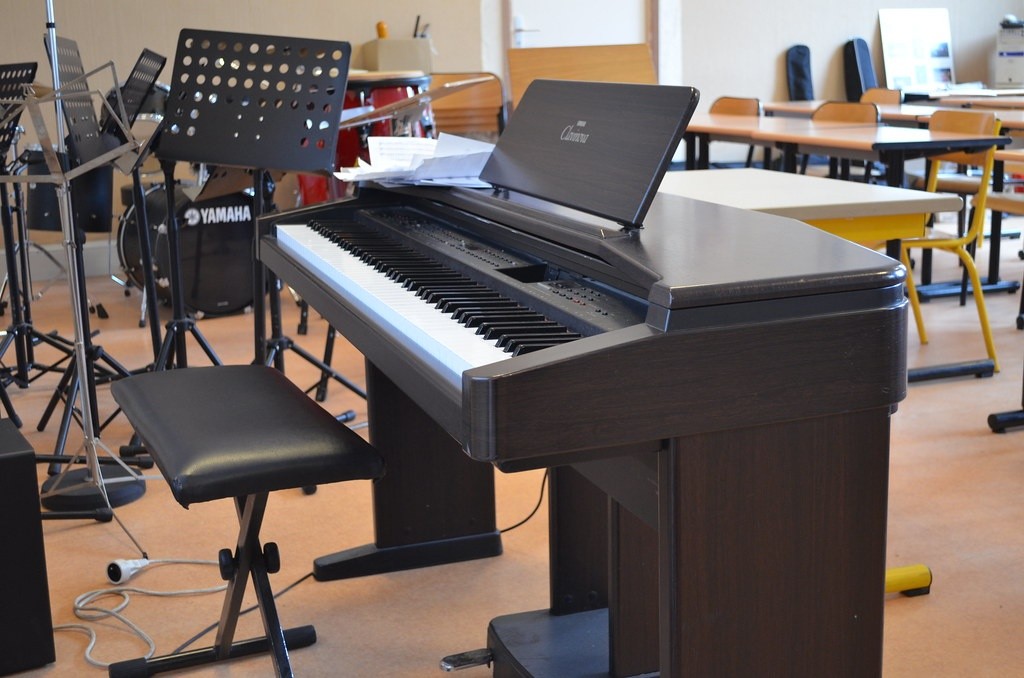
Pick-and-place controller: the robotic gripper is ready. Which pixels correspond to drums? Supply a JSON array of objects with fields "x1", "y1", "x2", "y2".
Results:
[
  {"x1": 296, "y1": 69, "x2": 437, "y2": 206},
  {"x1": 117, "y1": 179, "x2": 283, "y2": 320},
  {"x1": 98, "y1": 80, "x2": 172, "y2": 175}
]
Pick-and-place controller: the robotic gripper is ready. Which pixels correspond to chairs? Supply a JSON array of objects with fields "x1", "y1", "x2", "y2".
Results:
[
  {"x1": 880, "y1": 110, "x2": 1000, "y2": 374},
  {"x1": 797, "y1": 88, "x2": 902, "y2": 185},
  {"x1": 800, "y1": 101, "x2": 880, "y2": 183},
  {"x1": 430, "y1": 72, "x2": 507, "y2": 138},
  {"x1": 696, "y1": 96, "x2": 761, "y2": 167}
]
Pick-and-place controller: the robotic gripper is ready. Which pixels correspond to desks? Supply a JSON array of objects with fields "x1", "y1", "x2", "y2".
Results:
[{"x1": 657, "y1": 89, "x2": 1024, "y2": 383}]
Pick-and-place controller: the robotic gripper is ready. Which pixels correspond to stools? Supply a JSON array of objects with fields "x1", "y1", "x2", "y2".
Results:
[{"x1": 109, "y1": 364, "x2": 386, "y2": 678}]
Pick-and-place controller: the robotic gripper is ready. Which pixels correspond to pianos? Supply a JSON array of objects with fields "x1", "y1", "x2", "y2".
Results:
[{"x1": 254, "y1": 175, "x2": 909, "y2": 678}]
